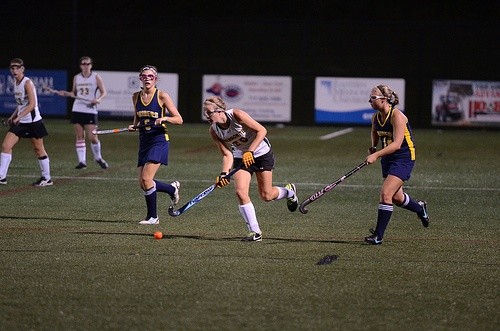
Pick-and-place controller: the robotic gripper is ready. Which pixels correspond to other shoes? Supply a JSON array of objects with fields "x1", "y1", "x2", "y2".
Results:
[
  {"x1": 241, "y1": 232, "x2": 263, "y2": 241},
  {"x1": 74, "y1": 162, "x2": 87, "y2": 169},
  {"x1": 32, "y1": 176, "x2": 53, "y2": 187},
  {"x1": 365, "y1": 228, "x2": 384, "y2": 246},
  {"x1": 96, "y1": 158, "x2": 109, "y2": 169},
  {"x1": 0, "y1": 177, "x2": 8, "y2": 185},
  {"x1": 285, "y1": 182, "x2": 299, "y2": 212},
  {"x1": 171, "y1": 180, "x2": 181, "y2": 205},
  {"x1": 138, "y1": 215, "x2": 159, "y2": 225},
  {"x1": 417, "y1": 199, "x2": 431, "y2": 228}
]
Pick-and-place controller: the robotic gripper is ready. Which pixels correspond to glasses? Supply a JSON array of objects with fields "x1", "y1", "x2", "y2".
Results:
[
  {"x1": 368, "y1": 95, "x2": 390, "y2": 101},
  {"x1": 81, "y1": 63, "x2": 92, "y2": 65},
  {"x1": 204, "y1": 110, "x2": 222, "y2": 117},
  {"x1": 140, "y1": 75, "x2": 155, "y2": 80}
]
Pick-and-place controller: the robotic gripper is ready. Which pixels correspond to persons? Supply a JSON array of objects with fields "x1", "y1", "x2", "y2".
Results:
[
  {"x1": 203, "y1": 96, "x2": 299, "y2": 242},
  {"x1": 0, "y1": 58, "x2": 53, "y2": 187},
  {"x1": 59, "y1": 56, "x2": 108, "y2": 169},
  {"x1": 127, "y1": 65, "x2": 183, "y2": 224},
  {"x1": 364, "y1": 85, "x2": 430, "y2": 245}
]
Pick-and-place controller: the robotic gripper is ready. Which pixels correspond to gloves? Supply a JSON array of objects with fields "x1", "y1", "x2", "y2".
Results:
[
  {"x1": 242, "y1": 150, "x2": 255, "y2": 168},
  {"x1": 216, "y1": 171, "x2": 230, "y2": 188}
]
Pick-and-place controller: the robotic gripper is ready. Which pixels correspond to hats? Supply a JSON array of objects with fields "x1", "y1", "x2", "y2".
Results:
[{"x1": 10, "y1": 58, "x2": 24, "y2": 66}]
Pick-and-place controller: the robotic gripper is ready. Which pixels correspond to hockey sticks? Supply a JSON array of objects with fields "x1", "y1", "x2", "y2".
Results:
[
  {"x1": 46, "y1": 86, "x2": 101, "y2": 105},
  {"x1": 2, "y1": 118, "x2": 20, "y2": 126},
  {"x1": 299, "y1": 161, "x2": 368, "y2": 214},
  {"x1": 168, "y1": 163, "x2": 244, "y2": 217},
  {"x1": 92, "y1": 120, "x2": 167, "y2": 135}
]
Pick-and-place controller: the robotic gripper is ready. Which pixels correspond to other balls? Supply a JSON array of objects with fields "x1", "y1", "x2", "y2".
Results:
[{"x1": 154, "y1": 231, "x2": 163, "y2": 239}]
